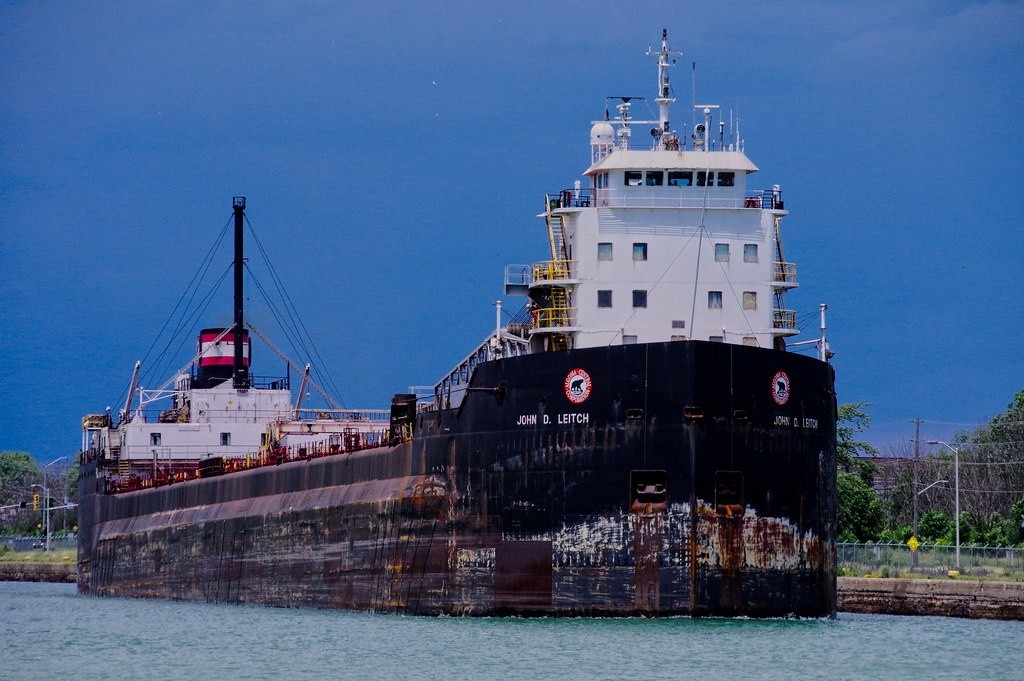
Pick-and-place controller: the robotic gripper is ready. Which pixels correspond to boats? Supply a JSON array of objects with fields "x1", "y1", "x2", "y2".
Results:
[
  {"x1": 74, "y1": 24, "x2": 839, "y2": 620},
  {"x1": 30, "y1": 484, "x2": 50, "y2": 554}
]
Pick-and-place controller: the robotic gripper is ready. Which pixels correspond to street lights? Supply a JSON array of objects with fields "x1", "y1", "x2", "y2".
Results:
[
  {"x1": 41, "y1": 456, "x2": 67, "y2": 534},
  {"x1": 927, "y1": 440, "x2": 960, "y2": 568},
  {"x1": 915, "y1": 480, "x2": 950, "y2": 567}
]
